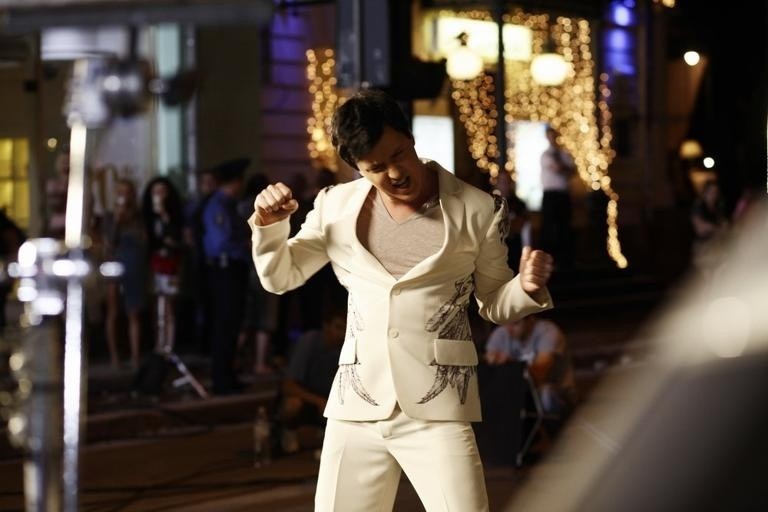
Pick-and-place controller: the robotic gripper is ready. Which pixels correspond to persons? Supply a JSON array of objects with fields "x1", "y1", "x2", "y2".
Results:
[
  {"x1": 0, "y1": 152, "x2": 348, "y2": 462},
  {"x1": 246, "y1": 88, "x2": 553, "y2": 511},
  {"x1": 686, "y1": 179, "x2": 763, "y2": 272},
  {"x1": 468, "y1": 124, "x2": 585, "y2": 461}
]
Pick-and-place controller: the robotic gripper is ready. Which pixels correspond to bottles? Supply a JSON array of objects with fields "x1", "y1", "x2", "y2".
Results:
[{"x1": 250, "y1": 403, "x2": 272, "y2": 468}]
